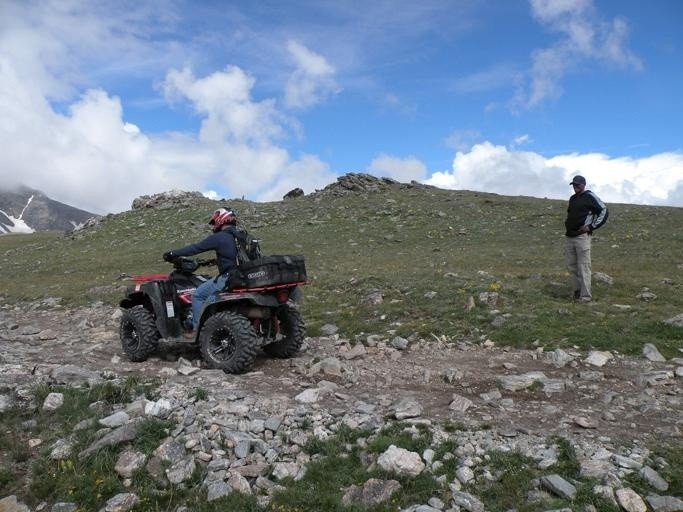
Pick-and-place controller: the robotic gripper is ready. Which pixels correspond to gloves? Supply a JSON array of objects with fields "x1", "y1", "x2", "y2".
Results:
[
  {"x1": 167, "y1": 250, "x2": 178, "y2": 260},
  {"x1": 207, "y1": 259, "x2": 217, "y2": 267}
]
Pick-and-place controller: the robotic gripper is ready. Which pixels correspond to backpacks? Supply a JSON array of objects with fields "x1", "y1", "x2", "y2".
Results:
[{"x1": 224, "y1": 227, "x2": 261, "y2": 266}]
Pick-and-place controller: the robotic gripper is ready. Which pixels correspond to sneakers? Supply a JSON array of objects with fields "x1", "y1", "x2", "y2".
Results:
[{"x1": 575, "y1": 290, "x2": 592, "y2": 302}]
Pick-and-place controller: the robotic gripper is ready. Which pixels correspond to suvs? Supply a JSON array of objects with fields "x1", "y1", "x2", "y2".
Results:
[{"x1": 115, "y1": 251, "x2": 310, "y2": 375}]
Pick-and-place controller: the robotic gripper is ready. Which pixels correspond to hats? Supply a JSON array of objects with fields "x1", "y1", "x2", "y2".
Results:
[{"x1": 570, "y1": 176, "x2": 586, "y2": 185}]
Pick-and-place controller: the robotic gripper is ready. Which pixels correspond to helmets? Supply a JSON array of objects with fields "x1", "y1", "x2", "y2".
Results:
[{"x1": 208, "y1": 207, "x2": 236, "y2": 233}]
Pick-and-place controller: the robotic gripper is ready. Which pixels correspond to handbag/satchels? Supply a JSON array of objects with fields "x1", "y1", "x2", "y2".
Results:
[{"x1": 226, "y1": 268, "x2": 246, "y2": 289}]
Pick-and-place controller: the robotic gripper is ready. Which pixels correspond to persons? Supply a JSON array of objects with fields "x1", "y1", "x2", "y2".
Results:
[
  {"x1": 163, "y1": 205, "x2": 248, "y2": 339},
  {"x1": 564, "y1": 175, "x2": 609, "y2": 303}
]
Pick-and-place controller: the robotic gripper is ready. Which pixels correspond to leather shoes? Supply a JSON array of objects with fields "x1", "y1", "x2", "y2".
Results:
[{"x1": 182, "y1": 331, "x2": 197, "y2": 339}]
